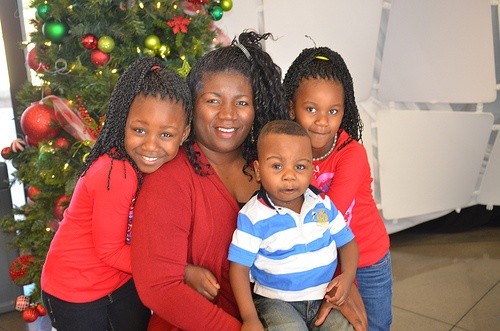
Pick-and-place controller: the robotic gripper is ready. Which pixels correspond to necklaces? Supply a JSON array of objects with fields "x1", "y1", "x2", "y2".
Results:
[{"x1": 312, "y1": 131, "x2": 337, "y2": 162}]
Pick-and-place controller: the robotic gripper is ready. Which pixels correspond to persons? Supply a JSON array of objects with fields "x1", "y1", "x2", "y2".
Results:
[{"x1": 40, "y1": 27, "x2": 392, "y2": 331}]
